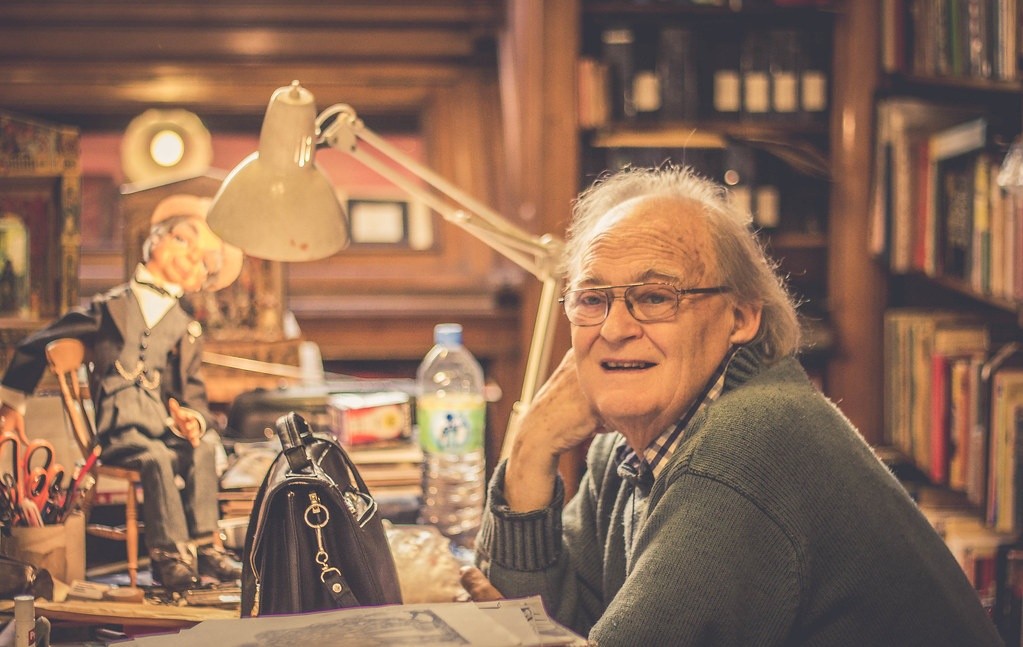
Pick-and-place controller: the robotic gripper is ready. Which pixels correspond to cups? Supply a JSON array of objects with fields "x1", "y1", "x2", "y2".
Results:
[{"x1": 0, "y1": 510, "x2": 87, "y2": 585}]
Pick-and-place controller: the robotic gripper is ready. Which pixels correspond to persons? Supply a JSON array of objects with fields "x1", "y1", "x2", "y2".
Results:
[
  {"x1": 477, "y1": 164, "x2": 1008, "y2": 647},
  {"x1": 0, "y1": 193, "x2": 246, "y2": 588}
]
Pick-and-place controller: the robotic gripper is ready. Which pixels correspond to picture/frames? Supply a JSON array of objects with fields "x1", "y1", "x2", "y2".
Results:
[{"x1": 0, "y1": 172, "x2": 69, "y2": 340}]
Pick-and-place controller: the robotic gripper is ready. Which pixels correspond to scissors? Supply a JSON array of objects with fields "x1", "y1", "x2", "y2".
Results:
[{"x1": 0, "y1": 431, "x2": 64, "y2": 526}]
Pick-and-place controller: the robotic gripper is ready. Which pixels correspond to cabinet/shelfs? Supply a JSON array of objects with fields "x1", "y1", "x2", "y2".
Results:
[{"x1": 572, "y1": 0, "x2": 1023, "y2": 647}]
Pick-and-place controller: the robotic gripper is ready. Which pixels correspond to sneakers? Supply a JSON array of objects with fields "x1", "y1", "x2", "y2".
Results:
[
  {"x1": 197, "y1": 548, "x2": 242, "y2": 581},
  {"x1": 152, "y1": 556, "x2": 196, "y2": 591}
]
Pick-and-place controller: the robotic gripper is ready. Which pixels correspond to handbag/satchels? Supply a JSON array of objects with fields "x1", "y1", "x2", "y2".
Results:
[{"x1": 240, "y1": 413, "x2": 402, "y2": 617}]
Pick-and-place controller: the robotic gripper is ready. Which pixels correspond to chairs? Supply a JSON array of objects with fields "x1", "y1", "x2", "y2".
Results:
[{"x1": 44, "y1": 336, "x2": 152, "y2": 593}]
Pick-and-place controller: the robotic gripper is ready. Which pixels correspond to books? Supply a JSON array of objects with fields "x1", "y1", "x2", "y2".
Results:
[{"x1": 578, "y1": 0, "x2": 1023, "y2": 646}]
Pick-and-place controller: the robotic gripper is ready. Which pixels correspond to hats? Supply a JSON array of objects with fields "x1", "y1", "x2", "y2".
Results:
[{"x1": 151, "y1": 195, "x2": 242, "y2": 291}]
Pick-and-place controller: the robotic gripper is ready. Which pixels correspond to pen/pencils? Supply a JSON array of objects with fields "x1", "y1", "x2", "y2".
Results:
[
  {"x1": 66, "y1": 464, "x2": 96, "y2": 519},
  {"x1": 71, "y1": 445, "x2": 102, "y2": 500},
  {"x1": 21, "y1": 498, "x2": 45, "y2": 527}
]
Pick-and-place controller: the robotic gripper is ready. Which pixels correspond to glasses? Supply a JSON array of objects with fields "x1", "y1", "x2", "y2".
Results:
[{"x1": 558, "y1": 283, "x2": 732, "y2": 327}]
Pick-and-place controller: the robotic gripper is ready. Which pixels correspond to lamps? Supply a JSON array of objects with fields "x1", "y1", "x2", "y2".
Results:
[{"x1": 202, "y1": 79, "x2": 575, "y2": 467}]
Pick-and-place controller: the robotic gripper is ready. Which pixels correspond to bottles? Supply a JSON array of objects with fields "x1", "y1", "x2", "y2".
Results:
[
  {"x1": 603, "y1": 26, "x2": 829, "y2": 124},
  {"x1": 416, "y1": 322, "x2": 486, "y2": 534},
  {"x1": 721, "y1": 141, "x2": 786, "y2": 231}
]
